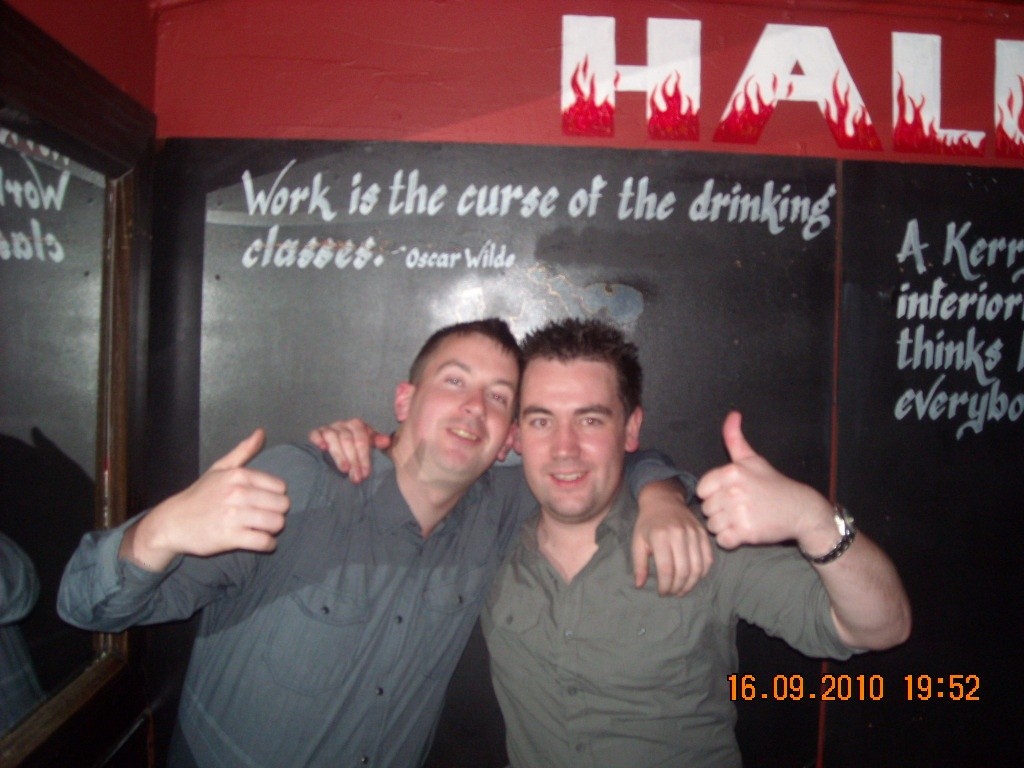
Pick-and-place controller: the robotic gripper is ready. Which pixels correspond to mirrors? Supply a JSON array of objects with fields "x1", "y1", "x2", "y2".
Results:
[{"x1": 0, "y1": 104, "x2": 138, "y2": 768}]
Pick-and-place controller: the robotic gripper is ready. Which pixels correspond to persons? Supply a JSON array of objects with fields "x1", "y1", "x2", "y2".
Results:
[{"x1": 56, "y1": 317, "x2": 911, "y2": 768}]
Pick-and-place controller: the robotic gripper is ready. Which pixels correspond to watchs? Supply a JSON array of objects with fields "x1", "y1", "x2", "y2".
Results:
[{"x1": 795, "y1": 500, "x2": 856, "y2": 565}]
